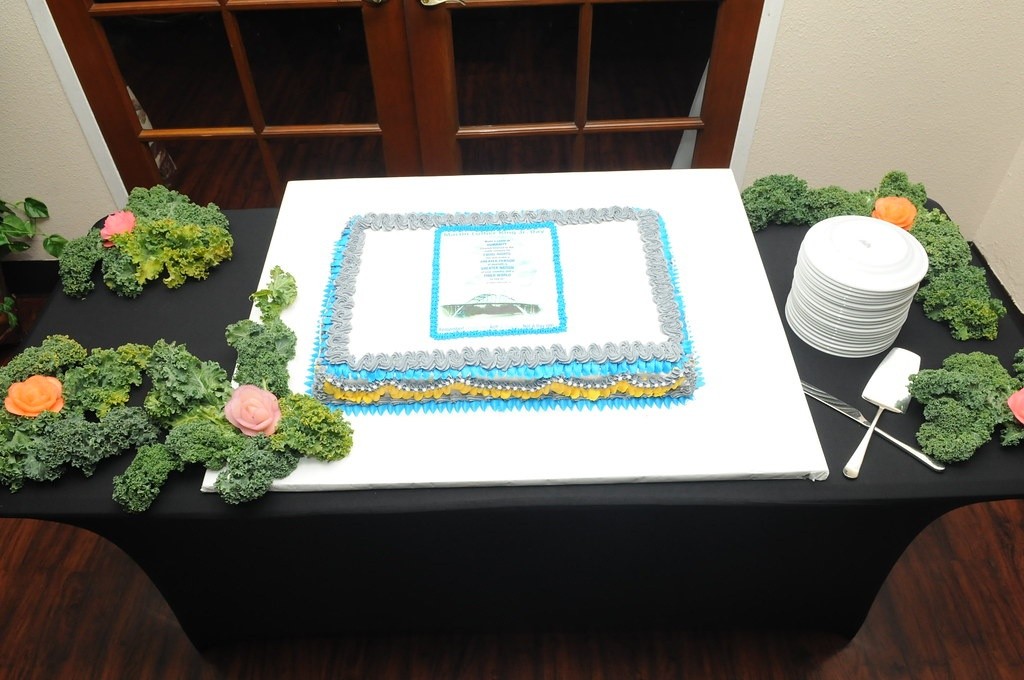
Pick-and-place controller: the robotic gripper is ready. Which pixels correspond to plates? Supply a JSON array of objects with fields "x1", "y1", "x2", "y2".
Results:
[{"x1": 783, "y1": 215, "x2": 930, "y2": 358}]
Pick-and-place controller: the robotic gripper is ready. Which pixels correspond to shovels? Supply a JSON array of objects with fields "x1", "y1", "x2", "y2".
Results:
[{"x1": 842, "y1": 347, "x2": 922, "y2": 479}]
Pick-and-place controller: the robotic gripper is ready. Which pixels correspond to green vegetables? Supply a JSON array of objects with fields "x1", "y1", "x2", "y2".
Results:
[
  {"x1": 905, "y1": 347, "x2": 1024, "y2": 464},
  {"x1": 0, "y1": 265, "x2": 354, "y2": 514},
  {"x1": 739, "y1": 170, "x2": 1007, "y2": 341},
  {"x1": 57, "y1": 184, "x2": 234, "y2": 299}
]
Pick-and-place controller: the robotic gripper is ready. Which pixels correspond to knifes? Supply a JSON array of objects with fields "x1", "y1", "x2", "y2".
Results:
[{"x1": 803, "y1": 379, "x2": 945, "y2": 473}]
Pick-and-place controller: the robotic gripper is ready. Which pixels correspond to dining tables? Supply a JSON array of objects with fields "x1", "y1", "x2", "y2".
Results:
[{"x1": 0, "y1": 192, "x2": 1023, "y2": 679}]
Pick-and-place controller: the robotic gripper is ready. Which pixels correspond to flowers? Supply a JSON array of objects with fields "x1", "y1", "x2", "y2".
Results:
[
  {"x1": 1007, "y1": 386, "x2": 1024, "y2": 424},
  {"x1": 870, "y1": 194, "x2": 917, "y2": 232},
  {"x1": 101, "y1": 209, "x2": 135, "y2": 249},
  {"x1": 3, "y1": 374, "x2": 65, "y2": 417},
  {"x1": 224, "y1": 383, "x2": 285, "y2": 441}
]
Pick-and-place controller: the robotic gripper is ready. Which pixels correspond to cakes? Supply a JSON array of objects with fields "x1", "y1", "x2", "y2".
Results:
[{"x1": 304, "y1": 204, "x2": 706, "y2": 417}]
